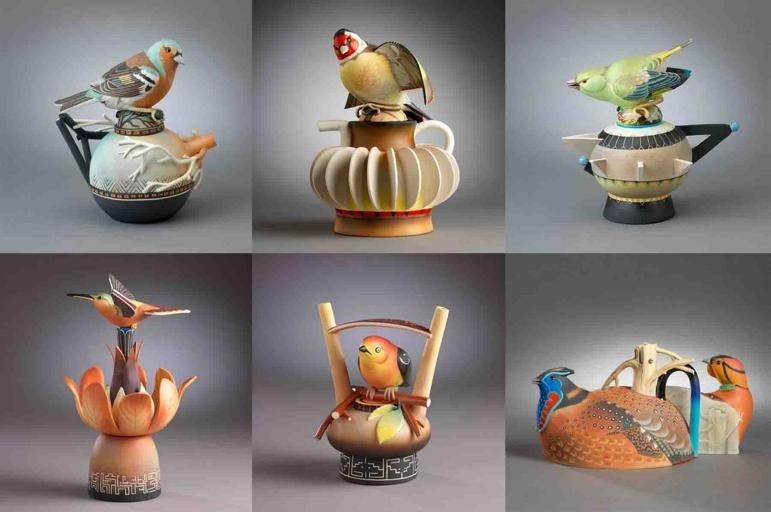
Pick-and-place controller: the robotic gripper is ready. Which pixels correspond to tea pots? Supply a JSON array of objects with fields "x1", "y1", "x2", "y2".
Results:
[
  {"x1": 563, "y1": 121, "x2": 735, "y2": 225},
  {"x1": 56, "y1": 110, "x2": 216, "y2": 224},
  {"x1": 310, "y1": 119, "x2": 460, "y2": 236}
]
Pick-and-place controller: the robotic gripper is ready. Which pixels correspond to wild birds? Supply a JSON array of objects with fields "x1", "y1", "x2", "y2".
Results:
[
  {"x1": 52, "y1": 37, "x2": 186, "y2": 123},
  {"x1": 332, "y1": 28, "x2": 435, "y2": 123},
  {"x1": 528, "y1": 366, "x2": 696, "y2": 469},
  {"x1": 565, "y1": 39, "x2": 695, "y2": 119},
  {"x1": 65, "y1": 273, "x2": 192, "y2": 333},
  {"x1": 700, "y1": 355, "x2": 754, "y2": 444},
  {"x1": 357, "y1": 336, "x2": 413, "y2": 402}
]
